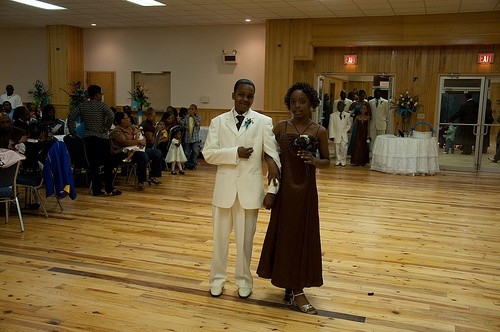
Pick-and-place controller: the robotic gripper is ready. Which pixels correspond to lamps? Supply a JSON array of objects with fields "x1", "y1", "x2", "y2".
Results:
[{"x1": 222, "y1": 49, "x2": 238, "y2": 64}]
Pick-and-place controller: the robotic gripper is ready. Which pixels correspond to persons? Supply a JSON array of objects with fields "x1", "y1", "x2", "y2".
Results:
[
  {"x1": 157, "y1": 106, "x2": 179, "y2": 125},
  {"x1": 123, "y1": 105, "x2": 136, "y2": 126},
  {"x1": 265, "y1": 82, "x2": 330, "y2": 314},
  {"x1": 488, "y1": 129, "x2": 500, "y2": 163},
  {"x1": 202, "y1": 78, "x2": 282, "y2": 298},
  {"x1": 447, "y1": 94, "x2": 478, "y2": 154},
  {"x1": 66, "y1": 85, "x2": 122, "y2": 196},
  {"x1": 0, "y1": 85, "x2": 65, "y2": 165},
  {"x1": 444, "y1": 121, "x2": 456, "y2": 154},
  {"x1": 138, "y1": 108, "x2": 159, "y2": 134},
  {"x1": 165, "y1": 129, "x2": 190, "y2": 175},
  {"x1": 324, "y1": 90, "x2": 392, "y2": 166},
  {"x1": 110, "y1": 112, "x2": 163, "y2": 188},
  {"x1": 180, "y1": 104, "x2": 200, "y2": 171},
  {"x1": 482, "y1": 98, "x2": 493, "y2": 153}
]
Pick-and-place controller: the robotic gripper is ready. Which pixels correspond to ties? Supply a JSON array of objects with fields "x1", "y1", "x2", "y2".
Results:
[
  {"x1": 340, "y1": 113, "x2": 342, "y2": 120},
  {"x1": 235, "y1": 115, "x2": 244, "y2": 130},
  {"x1": 376, "y1": 100, "x2": 378, "y2": 108}
]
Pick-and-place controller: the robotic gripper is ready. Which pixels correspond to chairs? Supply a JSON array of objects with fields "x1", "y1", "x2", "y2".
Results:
[{"x1": 0, "y1": 125, "x2": 183, "y2": 232}]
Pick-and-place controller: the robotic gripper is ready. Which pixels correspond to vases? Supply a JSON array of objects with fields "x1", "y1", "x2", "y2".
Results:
[{"x1": 402, "y1": 111, "x2": 412, "y2": 136}]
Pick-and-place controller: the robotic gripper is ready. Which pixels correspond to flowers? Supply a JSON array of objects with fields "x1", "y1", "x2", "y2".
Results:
[
  {"x1": 288, "y1": 135, "x2": 320, "y2": 175},
  {"x1": 389, "y1": 91, "x2": 422, "y2": 115},
  {"x1": 243, "y1": 118, "x2": 255, "y2": 130},
  {"x1": 128, "y1": 80, "x2": 151, "y2": 107}
]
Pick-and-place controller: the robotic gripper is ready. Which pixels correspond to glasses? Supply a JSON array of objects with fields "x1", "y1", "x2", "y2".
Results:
[
  {"x1": 96, "y1": 92, "x2": 104, "y2": 96},
  {"x1": 122, "y1": 117, "x2": 131, "y2": 120}
]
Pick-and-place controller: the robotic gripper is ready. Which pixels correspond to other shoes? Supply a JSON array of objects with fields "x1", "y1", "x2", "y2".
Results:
[
  {"x1": 137, "y1": 182, "x2": 144, "y2": 188},
  {"x1": 180, "y1": 170, "x2": 185, "y2": 174},
  {"x1": 171, "y1": 171, "x2": 177, "y2": 175},
  {"x1": 488, "y1": 157, "x2": 497, "y2": 163},
  {"x1": 106, "y1": 187, "x2": 115, "y2": 195},
  {"x1": 336, "y1": 161, "x2": 340, "y2": 166},
  {"x1": 93, "y1": 191, "x2": 103, "y2": 195},
  {"x1": 341, "y1": 162, "x2": 345, "y2": 166},
  {"x1": 461, "y1": 151, "x2": 470, "y2": 154},
  {"x1": 443, "y1": 151, "x2": 448, "y2": 154},
  {"x1": 148, "y1": 177, "x2": 161, "y2": 184}
]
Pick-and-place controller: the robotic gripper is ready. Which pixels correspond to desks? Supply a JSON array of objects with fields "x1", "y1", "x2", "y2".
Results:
[
  {"x1": 198, "y1": 126, "x2": 211, "y2": 143},
  {"x1": 371, "y1": 134, "x2": 439, "y2": 177}
]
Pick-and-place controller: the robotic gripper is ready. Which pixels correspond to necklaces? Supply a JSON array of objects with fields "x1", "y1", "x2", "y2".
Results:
[{"x1": 291, "y1": 119, "x2": 314, "y2": 146}]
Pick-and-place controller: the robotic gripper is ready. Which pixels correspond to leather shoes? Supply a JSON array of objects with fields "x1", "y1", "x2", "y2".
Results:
[
  {"x1": 211, "y1": 286, "x2": 222, "y2": 296},
  {"x1": 239, "y1": 287, "x2": 251, "y2": 298}
]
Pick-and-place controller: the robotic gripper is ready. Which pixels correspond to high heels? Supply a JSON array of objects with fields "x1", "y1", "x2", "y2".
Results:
[
  {"x1": 283, "y1": 286, "x2": 294, "y2": 306},
  {"x1": 289, "y1": 289, "x2": 319, "y2": 315}
]
